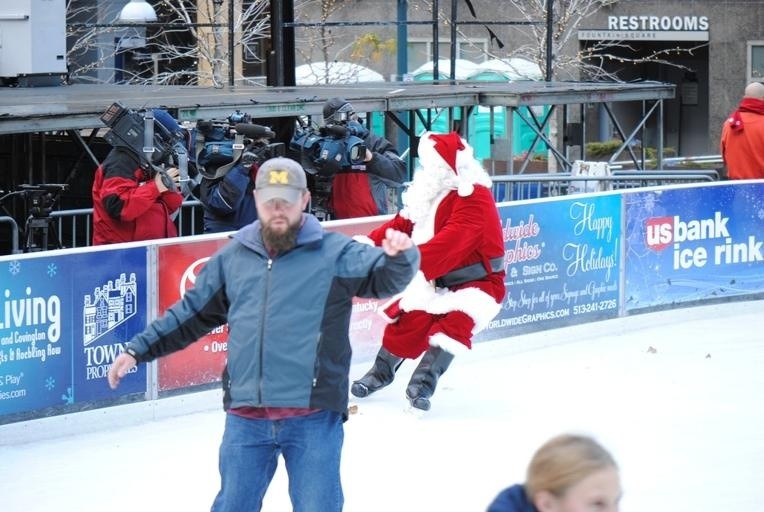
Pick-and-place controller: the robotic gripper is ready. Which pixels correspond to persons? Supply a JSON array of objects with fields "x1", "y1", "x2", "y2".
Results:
[
  {"x1": 89, "y1": 101, "x2": 184, "y2": 245},
  {"x1": 485, "y1": 431, "x2": 624, "y2": 512},
  {"x1": 349, "y1": 127, "x2": 510, "y2": 415},
  {"x1": 720, "y1": 82, "x2": 764, "y2": 180},
  {"x1": 199, "y1": 107, "x2": 273, "y2": 232},
  {"x1": 108, "y1": 156, "x2": 422, "y2": 512},
  {"x1": 304, "y1": 97, "x2": 406, "y2": 221}
]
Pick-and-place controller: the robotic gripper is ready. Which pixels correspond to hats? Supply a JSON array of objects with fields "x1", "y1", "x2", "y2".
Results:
[
  {"x1": 323, "y1": 97, "x2": 356, "y2": 125},
  {"x1": 255, "y1": 156, "x2": 306, "y2": 208},
  {"x1": 743, "y1": 82, "x2": 764, "y2": 97}
]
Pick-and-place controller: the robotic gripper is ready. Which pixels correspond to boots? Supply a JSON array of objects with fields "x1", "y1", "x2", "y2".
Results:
[
  {"x1": 406, "y1": 346, "x2": 454, "y2": 413},
  {"x1": 351, "y1": 346, "x2": 404, "y2": 398}
]
